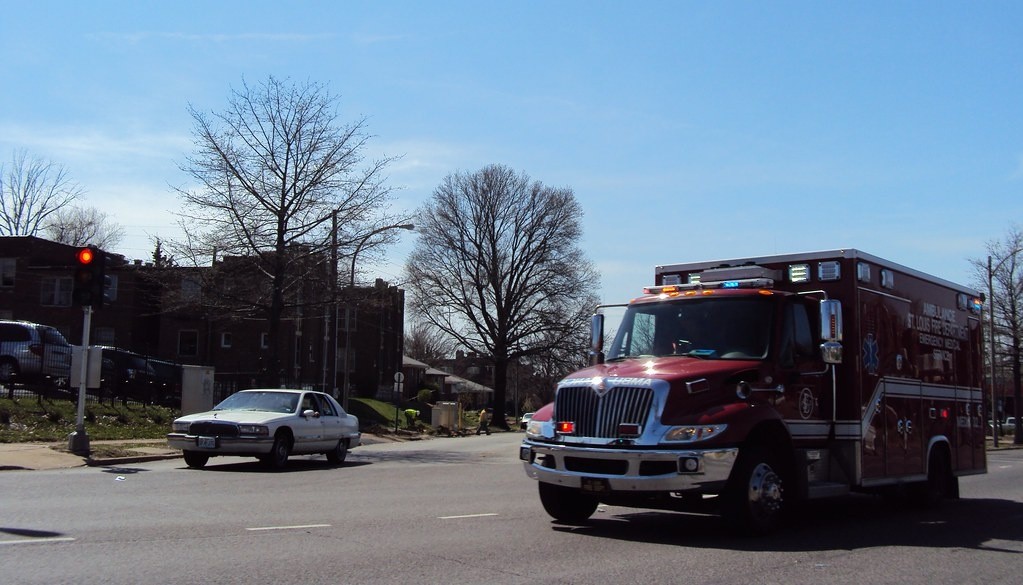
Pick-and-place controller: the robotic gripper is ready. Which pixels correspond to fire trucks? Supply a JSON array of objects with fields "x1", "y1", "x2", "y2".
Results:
[{"x1": 519, "y1": 247, "x2": 993, "y2": 535}]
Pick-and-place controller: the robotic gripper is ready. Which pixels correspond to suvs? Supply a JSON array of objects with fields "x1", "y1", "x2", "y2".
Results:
[
  {"x1": 90, "y1": 345, "x2": 180, "y2": 406},
  {"x1": 0, "y1": 318, "x2": 72, "y2": 397}
]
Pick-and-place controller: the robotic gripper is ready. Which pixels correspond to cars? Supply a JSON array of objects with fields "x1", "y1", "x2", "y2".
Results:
[
  {"x1": 167, "y1": 389, "x2": 361, "y2": 470},
  {"x1": 518, "y1": 413, "x2": 537, "y2": 430},
  {"x1": 989, "y1": 416, "x2": 1017, "y2": 435}
]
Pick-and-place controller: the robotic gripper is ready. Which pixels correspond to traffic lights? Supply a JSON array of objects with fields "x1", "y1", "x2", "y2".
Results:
[
  {"x1": 77, "y1": 245, "x2": 103, "y2": 309},
  {"x1": 98, "y1": 252, "x2": 114, "y2": 308}
]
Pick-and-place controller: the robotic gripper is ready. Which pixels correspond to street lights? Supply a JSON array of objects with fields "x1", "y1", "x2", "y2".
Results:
[{"x1": 344, "y1": 223, "x2": 414, "y2": 292}]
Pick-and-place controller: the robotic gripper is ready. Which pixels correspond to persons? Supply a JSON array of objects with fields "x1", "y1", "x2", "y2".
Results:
[
  {"x1": 475, "y1": 407, "x2": 491, "y2": 435},
  {"x1": 290, "y1": 397, "x2": 320, "y2": 417}
]
